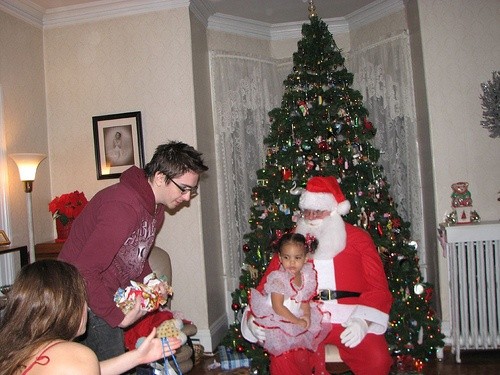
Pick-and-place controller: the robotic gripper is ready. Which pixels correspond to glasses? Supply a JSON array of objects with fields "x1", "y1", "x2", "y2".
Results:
[{"x1": 160, "y1": 172, "x2": 198, "y2": 193}]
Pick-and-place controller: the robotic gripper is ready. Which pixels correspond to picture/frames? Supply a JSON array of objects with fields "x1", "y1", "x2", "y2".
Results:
[
  {"x1": 91, "y1": 111, "x2": 145, "y2": 181},
  {"x1": 0, "y1": 229, "x2": 11, "y2": 245}
]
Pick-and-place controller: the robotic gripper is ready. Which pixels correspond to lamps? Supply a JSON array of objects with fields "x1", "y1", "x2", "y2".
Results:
[{"x1": 9, "y1": 153, "x2": 47, "y2": 263}]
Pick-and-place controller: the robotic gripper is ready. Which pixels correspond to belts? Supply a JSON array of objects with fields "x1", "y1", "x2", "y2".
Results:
[{"x1": 312, "y1": 289, "x2": 362, "y2": 302}]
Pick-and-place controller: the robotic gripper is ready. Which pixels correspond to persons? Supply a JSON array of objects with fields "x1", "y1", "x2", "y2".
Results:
[
  {"x1": 0, "y1": 259, "x2": 182, "y2": 375},
  {"x1": 240, "y1": 175, "x2": 394, "y2": 375},
  {"x1": 107, "y1": 132, "x2": 122, "y2": 165},
  {"x1": 248, "y1": 233, "x2": 333, "y2": 375},
  {"x1": 57, "y1": 139, "x2": 209, "y2": 375}
]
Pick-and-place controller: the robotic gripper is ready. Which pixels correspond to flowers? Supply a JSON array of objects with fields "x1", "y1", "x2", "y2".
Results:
[{"x1": 49, "y1": 190, "x2": 89, "y2": 221}]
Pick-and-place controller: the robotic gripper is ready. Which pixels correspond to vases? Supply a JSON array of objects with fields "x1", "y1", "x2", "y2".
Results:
[{"x1": 55, "y1": 217, "x2": 77, "y2": 243}]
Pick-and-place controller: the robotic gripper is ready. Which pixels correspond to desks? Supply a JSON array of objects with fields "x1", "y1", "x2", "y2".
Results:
[{"x1": 28, "y1": 242, "x2": 68, "y2": 260}]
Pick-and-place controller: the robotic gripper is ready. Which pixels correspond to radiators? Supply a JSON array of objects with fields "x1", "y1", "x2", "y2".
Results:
[{"x1": 440, "y1": 221, "x2": 500, "y2": 363}]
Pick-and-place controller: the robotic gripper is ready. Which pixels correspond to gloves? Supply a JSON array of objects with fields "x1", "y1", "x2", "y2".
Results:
[{"x1": 340, "y1": 318, "x2": 369, "y2": 348}]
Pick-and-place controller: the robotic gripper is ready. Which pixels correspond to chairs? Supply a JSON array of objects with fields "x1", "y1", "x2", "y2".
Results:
[{"x1": 147, "y1": 246, "x2": 196, "y2": 375}]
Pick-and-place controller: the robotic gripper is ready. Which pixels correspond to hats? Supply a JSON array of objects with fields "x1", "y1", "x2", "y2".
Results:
[{"x1": 298, "y1": 176, "x2": 351, "y2": 215}]
